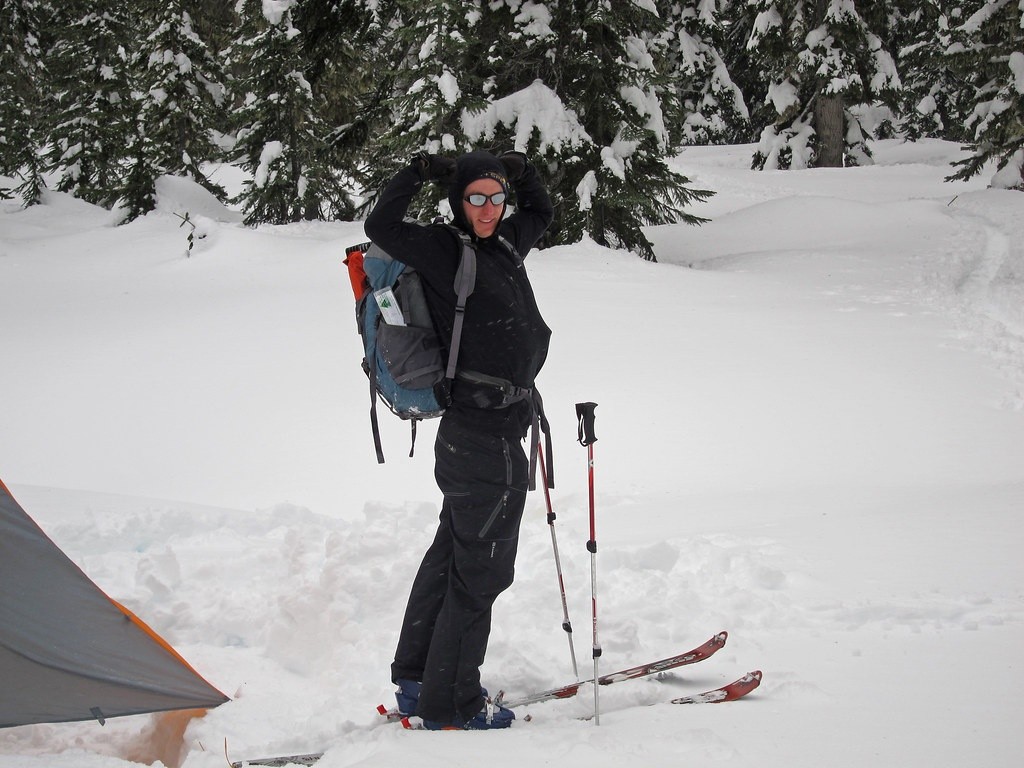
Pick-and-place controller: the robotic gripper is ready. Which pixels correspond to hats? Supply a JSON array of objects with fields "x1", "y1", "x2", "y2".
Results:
[{"x1": 480, "y1": 168, "x2": 512, "y2": 200}]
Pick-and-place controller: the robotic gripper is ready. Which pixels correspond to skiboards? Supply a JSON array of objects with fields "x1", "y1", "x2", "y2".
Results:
[{"x1": 225, "y1": 629, "x2": 765, "y2": 766}]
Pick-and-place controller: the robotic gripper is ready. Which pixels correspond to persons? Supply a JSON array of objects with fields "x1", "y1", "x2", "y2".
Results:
[{"x1": 363, "y1": 150, "x2": 554, "y2": 729}]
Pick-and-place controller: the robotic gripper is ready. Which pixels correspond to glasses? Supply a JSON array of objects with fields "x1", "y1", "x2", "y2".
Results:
[{"x1": 463, "y1": 192, "x2": 508, "y2": 207}]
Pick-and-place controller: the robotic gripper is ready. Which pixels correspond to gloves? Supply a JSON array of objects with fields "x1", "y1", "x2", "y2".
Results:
[
  {"x1": 410, "y1": 150, "x2": 458, "y2": 191},
  {"x1": 498, "y1": 150, "x2": 533, "y2": 188}
]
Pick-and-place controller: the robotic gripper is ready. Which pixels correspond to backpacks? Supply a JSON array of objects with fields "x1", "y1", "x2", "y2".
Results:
[{"x1": 342, "y1": 215, "x2": 477, "y2": 464}]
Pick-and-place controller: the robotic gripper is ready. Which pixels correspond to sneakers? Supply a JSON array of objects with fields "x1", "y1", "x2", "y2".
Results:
[
  {"x1": 416, "y1": 689, "x2": 515, "y2": 731},
  {"x1": 395, "y1": 683, "x2": 488, "y2": 720}
]
